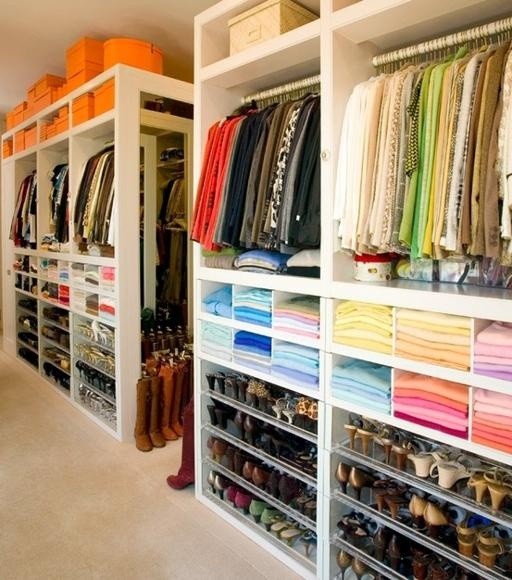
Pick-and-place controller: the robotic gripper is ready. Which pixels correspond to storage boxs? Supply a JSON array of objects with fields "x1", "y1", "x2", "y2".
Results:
[
  {"x1": 65, "y1": 36, "x2": 103, "y2": 92},
  {"x1": 39, "y1": 107, "x2": 69, "y2": 142},
  {"x1": 15, "y1": 127, "x2": 37, "y2": 154},
  {"x1": 103, "y1": 38, "x2": 165, "y2": 75},
  {"x1": 71, "y1": 79, "x2": 114, "y2": 127},
  {"x1": 228, "y1": 0, "x2": 319, "y2": 58},
  {"x1": 5, "y1": 73, "x2": 66, "y2": 130},
  {"x1": 3, "y1": 140, "x2": 12, "y2": 158}
]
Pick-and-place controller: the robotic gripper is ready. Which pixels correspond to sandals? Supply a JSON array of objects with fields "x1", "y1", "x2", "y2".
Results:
[
  {"x1": 456, "y1": 515, "x2": 482, "y2": 556},
  {"x1": 207, "y1": 396, "x2": 218, "y2": 426},
  {"x1": 344, "y1": 413, "x2": 359, "y2": 450},
  {"x1": 385, "y1": 483, "x2": 410, "y2": 518},
  {"x1": 356, "y1": 414, "x2": 378, "y2": 456},
  {"x1": 468, "y1": 463, "x2": 487, "y2": 503},
  {"x1": 394, "y1": 434, "x2": 431, "y2": 473},
  {"x1": 283, "y1": 397, "x2": 298, "y2": 424},
  {"x1": 216, "y1": 401, "x2": 235, "y2": 430},
  {"x1": 273, "y1": 394, "x2": 290, "y2": 419},
  {"x1": 430, "y1": 455, "x2": 480, "y2": 487},
  {"x1": 78, "y1": 384, "x2": 116, "y2": 426},
  {"x1": 373, "y1": 480, "x2": 385, "y2": 513},
  {"x1": 270, "y1": 514, "x2": 296, "y2": 539},
  {"x1": 77, "y1": 319, "x2": 115, "y2": 350},
  {"x1": 305, "y1": 494, "x2": 316, "y2": 518},
  {"x1": 378, "y1": 424, "x2": 400, "y2": 464},
  {"x1": 337, "y1": 509, "x2": 364, "y2": 541},
  {"x1": 408, "y1": 444, "x2": 448, "y2": 479},
  {"x1": 75, "y1": 343, "x2": 115, "y2": 375},
  {"x1": 280, "y1": 522, "x2": 312, "y2": 547},
  {"x1": 311, "y1": 533, "x2": 317, "y2": 546},
  {"x1": 484, "y1": 464, "x2": 512, "y2": 514},
  {"x1": 347, "y1": 518, "x2": 381, "y2": 550},
  {"x1": 299, "y1": 530, "x2": 312, "y2": 556},
  {"x1": 475, "y1": 524, "x2": 512, "y2": 568},
  {"x1": 298, "y1": 490, "x2": 313, "y2": 513}
]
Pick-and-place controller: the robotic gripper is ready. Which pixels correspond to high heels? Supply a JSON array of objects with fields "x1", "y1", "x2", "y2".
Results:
[
  {"x1": 262, "y1": 426, "x2": 273, "y2": 453},
  {"x1": 423, "y1": 501, "x2": 456, "y2": 539},
  {"x1": 226, "y1": 484, "x2": 246, "y2": 508},
  {"x1": 268, "y1": 471, "x2": 280, "y2": 498},
  {"x1": 76, "y1": 359, "x2": 115, "y2": 400},
  {"x1": 226, "y1": 446, "x2": 238, "y2": 471},
  {"x1": 279, "y1": 474, "x2": 299, "y2": 504},
  {"x1": 214, "y1": 440, "x2": 226, "y2": 463},
  {"x1": 351, "y1": 557, "x2": 374, "y2": 580},
  {"x1": 207, "y1": 435, "x2": 216, "y2": 459},
  {"x1": 244, "y1": 414, "x2": 258, "y2": 445},
  {"x1": 206, "y1": 371, "x2": 215, "y2": 390},
  {"x1": 207, "y1": 469, "x2": 220, "y2": 494},
  {"x1": 242, "y1": 460, "x2": 262, "y2": 481},
  {"x1": 251, "y1": 466, "x2": 269, "y2": 488},
  {"x1": 214, "y1": 474, "x2": 234, "y2": 500},
  {"x1": 336, "y1": 550, "x2": 352, "y2": 579},
  {"x1": 373, "y1": 527, "x2": 389, "y2": 563},
  {"x1": 272, "y1": 432, "x2": 306, "y2": 459},
  {"x1": 261, "y1": 506, "x2": 282, "y2": 532},
  {"x1": 334, "y1": 461, "x2": 349, "y2": 494},
  {"x1": 305, "y1": 401, "x2": 318, "y2": 433},
  {"x1": 348, "y1": 466, "x2": 376, "y2": 502},
  {"x1": 389, "y1": 535, "x2": 411, "y2": 573},
  {"x1": 237, "y1": 374, "x2": 248, "y2": 403},
  {"x1": 256, "y1": 383, "x2": 290, "y2": 412},
  {"x1": 410, "y1": 493, "x2": 428, "y2": 528},
  {"x1": 248, "y1": 378, "x2": 259, "y2": 407},
  {"x1": 226, "y1": 373, "x2": 237, "y2": 398},
  {"x1": 294, "y1": 397, "x2": 310, "y2": 427},
  {"x1": 249, "y1": 498, "x2": 272, "y2": 524},
  {"x1": 233, "y1": 452, "x2": 260, "y2": 475},
  {"x1": 214, "y1": 370, "x2": 227, "y2": 394},
  {"x1": 234, "y1": 411, "x2": 245, "y2": 439},
  {"x1": 235, "y1": 490, "x2": 259, "y2": 515}
]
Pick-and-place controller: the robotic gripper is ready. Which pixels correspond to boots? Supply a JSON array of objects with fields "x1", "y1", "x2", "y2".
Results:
[
  {"x1": 171, "y1": 366, "x2": 183, "y2": 437},
  {"x1": 159, "y1": 368, "x2": 178, "y2": 440},
  {"x1": 167, "y1": 396, "x2": 194, "y2": 490},
  {"x1": 149, "y1": 376, "x2": 166, "y2": 448},
  {"x1": 426, "y1": 556, "x2": 457, "y2": 580},
  {"x1": 134, "y1": 378, "x2": 153, "y2": 451},
  {"x1": 412, "y1": 549, "x2": 431, "y2": 580}
]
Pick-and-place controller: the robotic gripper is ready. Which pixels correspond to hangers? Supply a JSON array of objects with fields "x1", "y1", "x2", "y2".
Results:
[
  {"x1": 241, "y1": 75, "x2": 322, "y2": 111},
  {"x1": 372, "y1": 19, "x2": 512, "y2": 75}
]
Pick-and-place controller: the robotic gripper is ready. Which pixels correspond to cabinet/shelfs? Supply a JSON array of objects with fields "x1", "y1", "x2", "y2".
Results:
[
  {"x1": 193, "y1": 0, "x2": 512, "y2": 580},
  {"x1": 0, "y1": 64, "x2": 193, "y2": 442}
]
